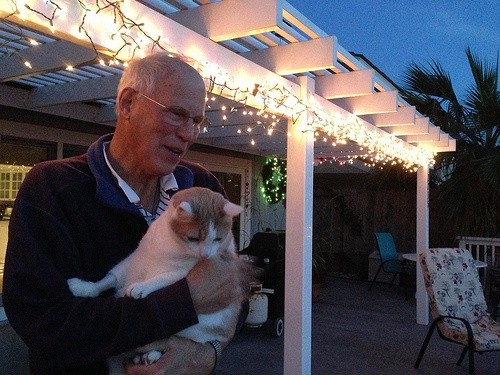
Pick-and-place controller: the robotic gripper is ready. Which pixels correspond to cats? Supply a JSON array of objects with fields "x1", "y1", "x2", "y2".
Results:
[{"x1": 67, "y1": 187, "x2": 244, "y2": 366}]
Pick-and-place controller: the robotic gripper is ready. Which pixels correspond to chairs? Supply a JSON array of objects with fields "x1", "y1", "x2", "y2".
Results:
[
  {"x1": 238, "y1": 233, "x2": 278, "y2": 274},
  {"x1": 414, "y1": 249, "x2": 500, "y2": 375},
  {"x1": 369, "y1": 232, "x2": 405, "y2": 291}
]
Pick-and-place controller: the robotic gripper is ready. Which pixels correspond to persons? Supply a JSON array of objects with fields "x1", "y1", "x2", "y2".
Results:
[{"x1": 2, "y1": 52, "x2": 265, "y2": 375}]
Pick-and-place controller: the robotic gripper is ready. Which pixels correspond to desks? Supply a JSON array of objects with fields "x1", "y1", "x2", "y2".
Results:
[{"x1": 399, "y1": 252, "x2": 488, "y2": 269}]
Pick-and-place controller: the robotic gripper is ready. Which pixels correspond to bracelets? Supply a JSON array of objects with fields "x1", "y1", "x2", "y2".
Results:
[{"x1": 208, "y1": 339, "x2": 222, "y2": 375}]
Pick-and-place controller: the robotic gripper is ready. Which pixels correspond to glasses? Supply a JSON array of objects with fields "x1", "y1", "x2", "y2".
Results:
[{"x1": 133, "y1": 89, "x2": 210, "y2": 134}]
